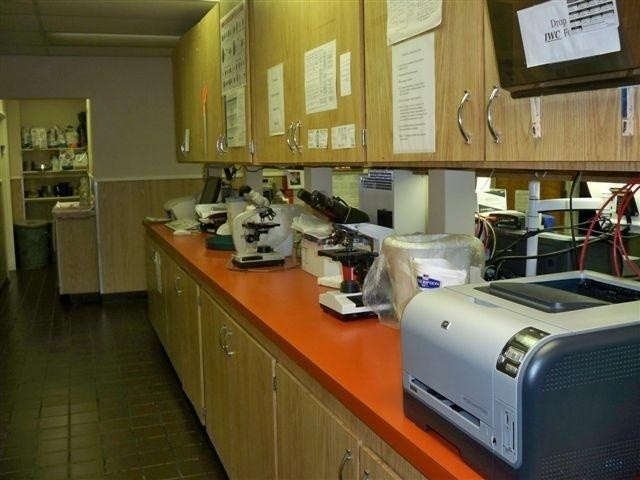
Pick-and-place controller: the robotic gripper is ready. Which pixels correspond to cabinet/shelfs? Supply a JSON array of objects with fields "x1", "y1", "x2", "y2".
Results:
[
  {"x1": 200, "y1": 289, "x2": 278, "y2": 479},
  {"x1": 205, "y1": 0, "x2": 252, "y2": 166},
  {"x1": 278, "y1": 359, "x2": 425, "y2": 479},
  {"x1": 173, "y1": 17, "x2": 206, "y2": 162},
  {"x1": 163, "y1": 252, "x2": 205, "y2": 425},
  {"x1": 364, "y1": 0, "x2": 640, "y2": 173},
  {"x1": 51, "y1": 200, "x2": 102, "y2": 295},
  {"x1": 140, "y1": 232, "x2": 165, "y2": 350},
  {"x1": 1, "y1": 97, "x2": 91, "y2": 219},
  {"x1": 250, "y1": 0, "x2": 366, "y2": 168}
]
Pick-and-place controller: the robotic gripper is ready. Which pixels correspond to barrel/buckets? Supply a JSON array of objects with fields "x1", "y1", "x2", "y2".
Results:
[
  {"x1": 30, "y1": 128, "x2": 48, "y2": 149},
  {"x1": 14, "y1": 218, "x2": 53, "y2": 270},
  {"x1": 382, "y1": 234, "x2": 470, "y2": 320}
]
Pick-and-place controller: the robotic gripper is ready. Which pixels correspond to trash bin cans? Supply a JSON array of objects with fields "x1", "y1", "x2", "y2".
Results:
[{"x1": 14, "y1": 219, "x2": 50, "y2": 270}]
